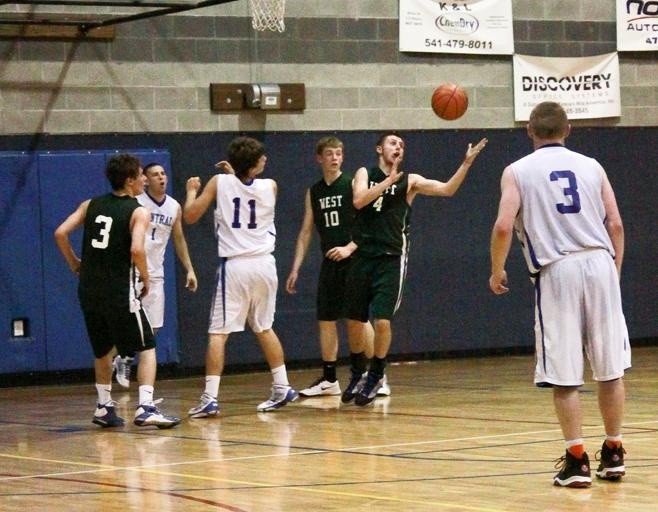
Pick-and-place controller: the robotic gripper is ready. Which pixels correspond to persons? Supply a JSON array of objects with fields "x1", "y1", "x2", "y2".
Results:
[
  {"x1": 286, "y1": 136, "x2": 390, "y2": 399},
  {"x1": 114, "y1": 162, "x2": 198, "y2": 389},
  {"x1": 54, "y1": 154, "x2": 182, "y2": 430},
  {"x1": 183, "y1": 137, "x2": 299, "y2": 419},
  {"x1": 341, "y1": 133, "x2": 488, "y2": 406},
  {"x1": 490, "y1": 103, "x2": 632, "y2": 489}
]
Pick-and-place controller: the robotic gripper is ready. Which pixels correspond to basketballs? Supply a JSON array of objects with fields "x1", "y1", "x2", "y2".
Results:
[{"x1": 432, "y1": 83, "x2": 468, "y2": 120}]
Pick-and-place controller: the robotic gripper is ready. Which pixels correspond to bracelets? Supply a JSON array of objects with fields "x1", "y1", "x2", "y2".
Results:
[{"x1": 464, "y1": 160, "x2": 472, "y2": 166}]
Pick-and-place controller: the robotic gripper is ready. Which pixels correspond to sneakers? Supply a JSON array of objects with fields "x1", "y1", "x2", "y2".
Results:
[
  {"x1": 92, "y1": 391, "x2": 220, "y2": 427},
  {"x1": 554, "y1": 451, "x2": 591, "y2": 488},
  {"x1": 257, "y1": 371, "x2": 389, "y2": 412},
  {"x1": 595, "y1": 441, "x2": 627, "y2": 479}
]
